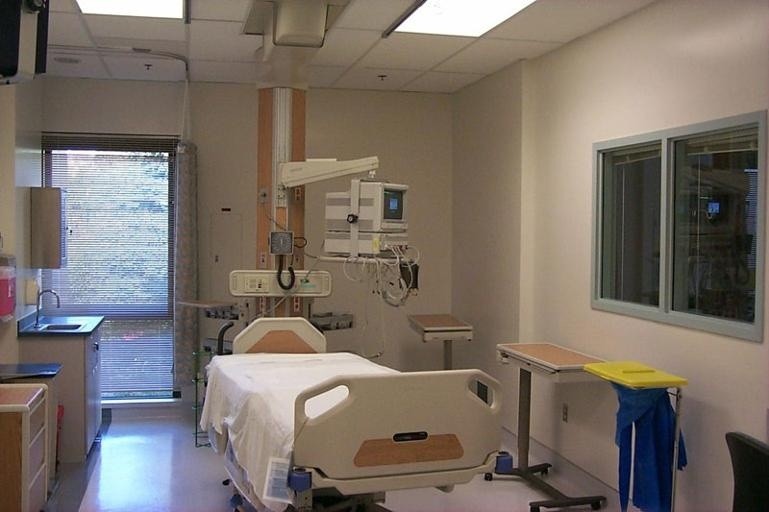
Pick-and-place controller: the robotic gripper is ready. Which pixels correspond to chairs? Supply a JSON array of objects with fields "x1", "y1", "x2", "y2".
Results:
[{"x1": 724, "y1": 432, "x2": 769, "y2": 511}]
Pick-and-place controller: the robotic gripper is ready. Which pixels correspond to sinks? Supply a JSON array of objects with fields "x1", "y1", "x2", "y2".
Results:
[{"x1": 41, "y1": 324, "x2": 81, "y2": 330}]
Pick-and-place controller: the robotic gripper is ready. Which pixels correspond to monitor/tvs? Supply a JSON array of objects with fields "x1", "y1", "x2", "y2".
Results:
[
  {"x1": 323, "y1": 180, "x2": 411, "y2": 233},
  {"x1": 704, "y1": 197, "x2": 726, "y2": 222}
]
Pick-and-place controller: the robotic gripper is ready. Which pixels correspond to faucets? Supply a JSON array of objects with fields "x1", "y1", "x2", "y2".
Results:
[{"x1": 36, "y1": 290, "x2": 60, "y2": 324}]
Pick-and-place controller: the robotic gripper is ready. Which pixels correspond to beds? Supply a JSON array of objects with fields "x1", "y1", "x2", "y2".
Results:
[{"x1": 198, "y1": 315, "x2": 506, "y2": 510}]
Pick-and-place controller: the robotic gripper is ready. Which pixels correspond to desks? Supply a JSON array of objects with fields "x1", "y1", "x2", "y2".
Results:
[
  {"x1": 486, "y1": 341, "x2": 608, "y2": 512},
  {"x1": 407, "y1": 315, "x2": 474, "y2": 370}
]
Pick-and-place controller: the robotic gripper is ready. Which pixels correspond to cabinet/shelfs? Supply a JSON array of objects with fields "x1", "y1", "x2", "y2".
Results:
[
  {"x1": 0, "y1": 382, "x2": 50, "y2": 512},
  {"x1": 30, "y1": 186, "x2": 69, "y2": 269},
  {"x1": 19, "y1": 327, "x2": 103, "y2": 462}
]
